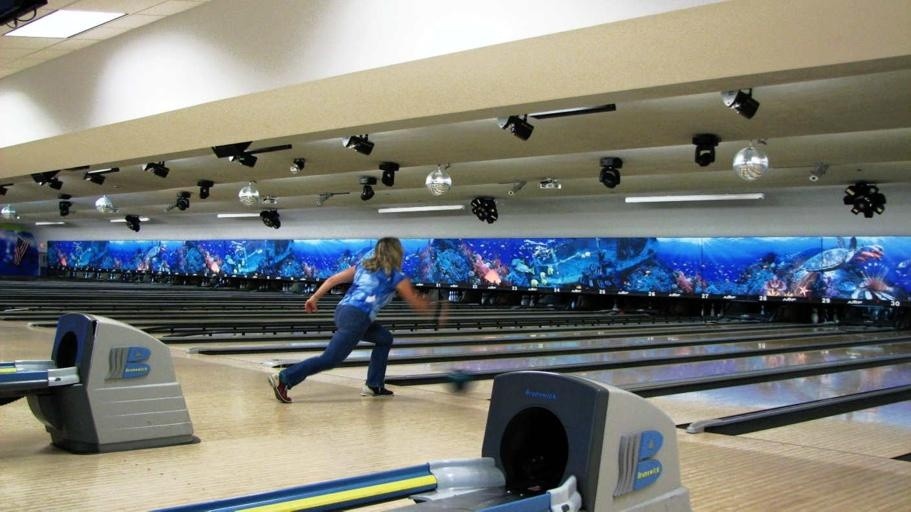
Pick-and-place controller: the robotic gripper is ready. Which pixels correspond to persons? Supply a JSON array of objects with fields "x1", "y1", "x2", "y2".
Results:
[{"x1": 266, "y1": 237, "x2": 447, "y2": 404}]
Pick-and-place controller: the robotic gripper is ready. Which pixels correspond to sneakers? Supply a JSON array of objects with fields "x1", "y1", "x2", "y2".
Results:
[
  {"x1": 361, "y1": 385, "x2": 393, "y2": 396},
  {"x1": 267, "y1": 373, "x2": 291, "y2": 403}
]
